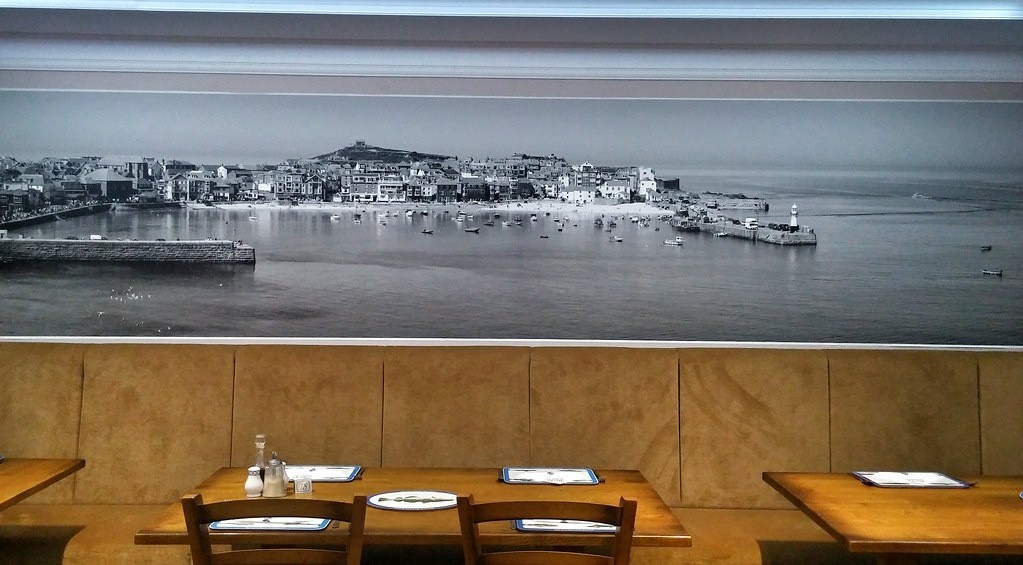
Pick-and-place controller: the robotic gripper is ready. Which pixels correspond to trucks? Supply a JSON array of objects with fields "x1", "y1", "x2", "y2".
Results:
[{"x1": 745, "y1": 218, "x2": 758, "y2": 229}]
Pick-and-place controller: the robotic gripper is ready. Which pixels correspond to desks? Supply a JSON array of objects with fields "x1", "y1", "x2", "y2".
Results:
[
  {"x1": 0, "y1": 457, "x2": 89, "y2": 515},
  {"x1": 134, "y1": 463, "x2": 695, "y2": 565},
  {"x1": 757, "y1": 461, "x2": 1023, "y2": 558}
]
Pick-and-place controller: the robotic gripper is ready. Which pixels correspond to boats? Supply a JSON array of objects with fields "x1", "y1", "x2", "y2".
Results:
[
  {"x1": 329, "y1": 209, "x2": 727, "y2": 246},
  {"x1": 982, "y1": 269, "x2": 1003, "y2": 275}
]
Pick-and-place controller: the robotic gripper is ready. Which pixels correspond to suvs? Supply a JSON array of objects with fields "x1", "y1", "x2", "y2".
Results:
[{"x1": 769, "y1": 223, "x2": 790, "y2": 231}]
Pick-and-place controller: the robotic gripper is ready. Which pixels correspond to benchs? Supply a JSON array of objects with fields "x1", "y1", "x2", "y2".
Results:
[{"x1": 0, "y1": 340, "x2": 1023, "y2": 565}]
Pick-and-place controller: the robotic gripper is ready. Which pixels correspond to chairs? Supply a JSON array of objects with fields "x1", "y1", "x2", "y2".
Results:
[
  {"x1": 179, "y1": 492, "x2": 366, "y2": 565},
  {"x1": 455, "y1": 492, "x2": 644, "y2": 565}
]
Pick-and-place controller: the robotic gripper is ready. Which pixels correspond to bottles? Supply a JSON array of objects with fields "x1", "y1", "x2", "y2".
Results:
[
  {"x1": 281, "y1": 462, "x2": 289, "y2": 485},
  {"x1": 262, "y1": 451, "x2": 286, "y2": 498},
  {"x1": 254, "y1": 434, "x2": 265, "y2": 480},
  {"x1": 244, "y1": 467, "x2": 264, "y2": 497}
]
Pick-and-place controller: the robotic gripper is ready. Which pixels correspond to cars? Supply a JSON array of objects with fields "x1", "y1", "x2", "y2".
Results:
[{"x1": 733, "y1": 220, "x2": 740, "y2": 224}]
